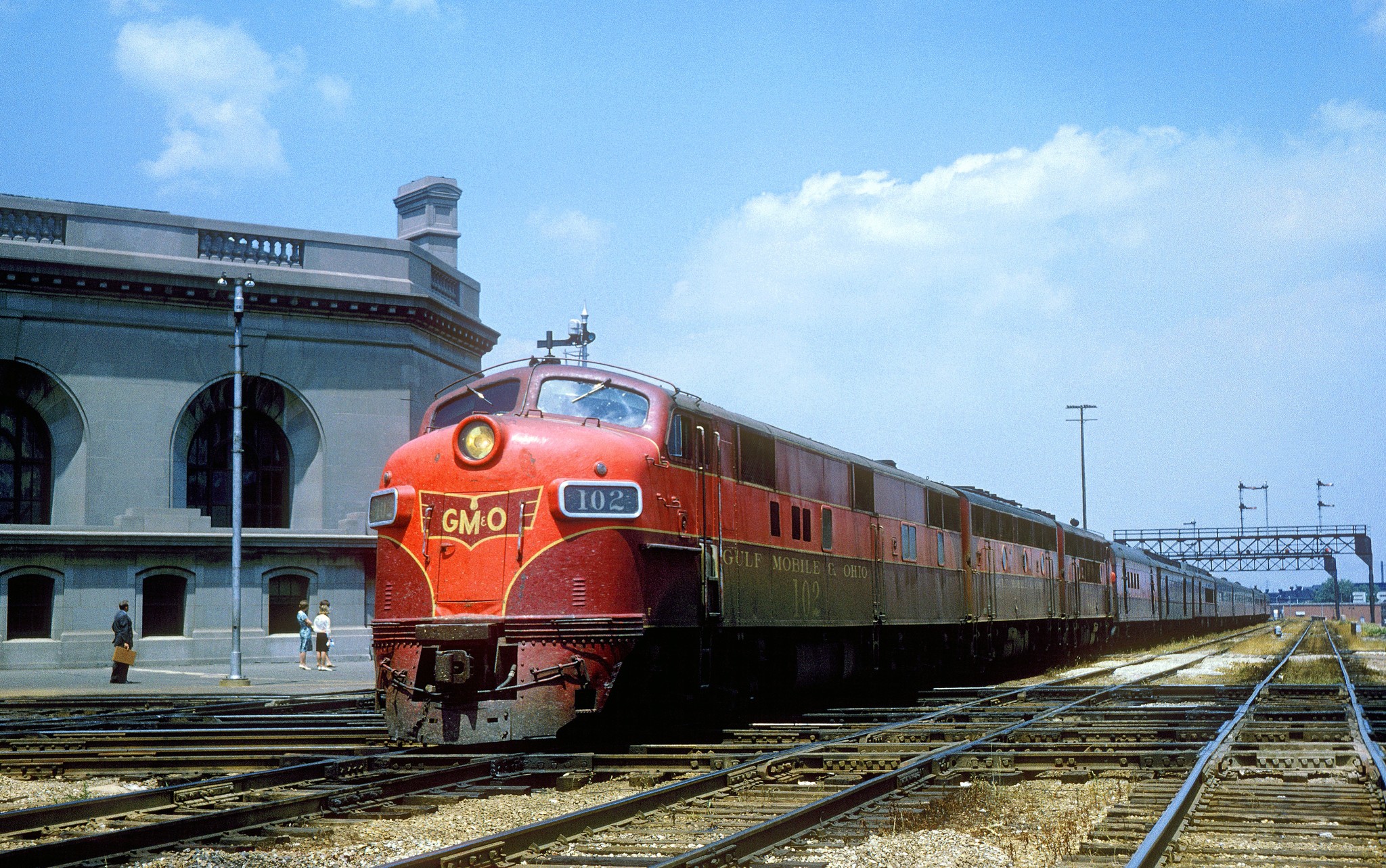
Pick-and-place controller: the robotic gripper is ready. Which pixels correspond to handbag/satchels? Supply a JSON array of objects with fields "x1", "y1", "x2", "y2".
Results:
[
  {"x1": 310, "y1": 624, "x2": 314, "y2": 632},
  {"x1": 328, "y1": 638, "x2": 335, "y2": 647}
]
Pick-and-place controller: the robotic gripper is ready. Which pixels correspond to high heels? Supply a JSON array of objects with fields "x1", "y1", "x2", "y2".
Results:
[{"x1": 299, "y1": 664, "x2": 311, "y2": 670}]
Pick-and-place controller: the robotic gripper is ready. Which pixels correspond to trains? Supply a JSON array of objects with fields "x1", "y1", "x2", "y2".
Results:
[{"x1": 362, "y1": 306, "x2": 1268, "y2": 751}]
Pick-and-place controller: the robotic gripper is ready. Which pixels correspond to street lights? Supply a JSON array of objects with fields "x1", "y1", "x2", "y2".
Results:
[{"x1": 217, "y1": 271, "x2": 256, "y2": 687}]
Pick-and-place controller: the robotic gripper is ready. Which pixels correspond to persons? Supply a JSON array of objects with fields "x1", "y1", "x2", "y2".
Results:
[
  {"x1": 316, "y1": 599, "x2": 337, "y2": 668},
  {"x1": 296, "y1": 599, "x2": 314, "y2": 670},
  {"x1": 313, "y1": 604, "x2": 334, "y2": 672},
  {"x1": 109, "y1": 599, "x2": 134, "y2": 684}
]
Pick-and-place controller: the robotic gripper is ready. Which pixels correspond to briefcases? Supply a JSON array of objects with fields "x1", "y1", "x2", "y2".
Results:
[{"x1": 112, "y1": 645, "x2": 136, "y2": 666}]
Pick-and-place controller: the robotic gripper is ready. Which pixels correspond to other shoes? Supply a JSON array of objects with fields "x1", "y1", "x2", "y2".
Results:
[
  {"x1": 110, "y1": 679, "x2": 133, "y2": 684},
  {"x1": 327, "y1": 663, "x2": 336, "y2": 667},
  {"x1": 318, "y1": 666, "x2": 333, "y2": 671}
]
